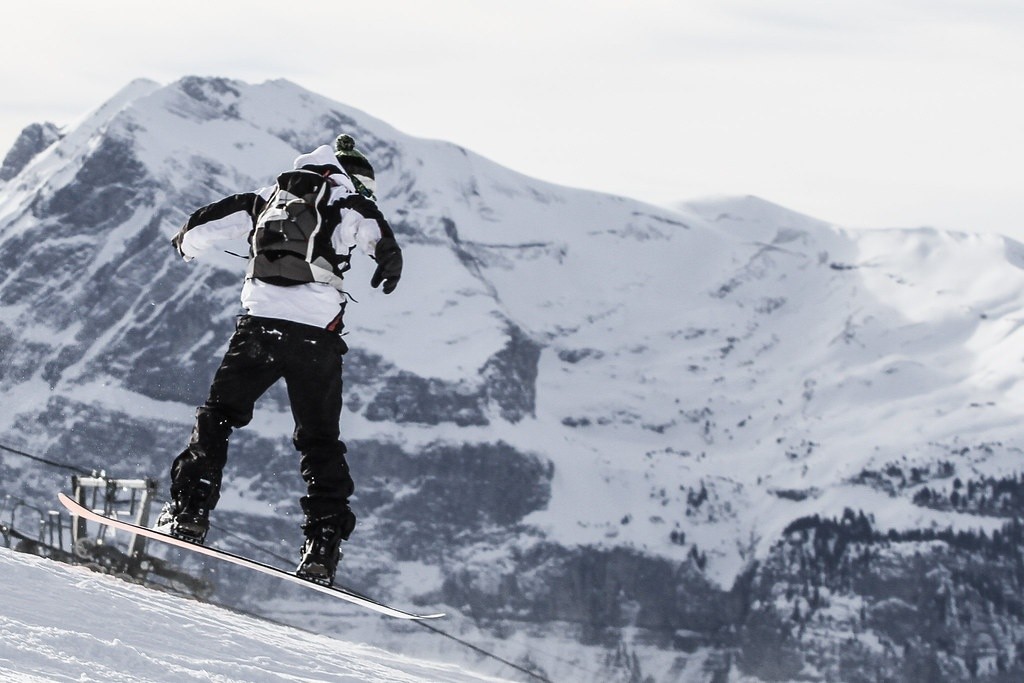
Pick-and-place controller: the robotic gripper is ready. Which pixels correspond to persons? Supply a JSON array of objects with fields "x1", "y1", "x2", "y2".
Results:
[{"x1": 154, "y1": 134, "x2": 403, "y2": 588}]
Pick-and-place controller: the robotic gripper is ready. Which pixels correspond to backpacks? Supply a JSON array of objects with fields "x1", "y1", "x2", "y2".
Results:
[{"x1": 249, "y1": 170, "x2": 348, "y2": 284}]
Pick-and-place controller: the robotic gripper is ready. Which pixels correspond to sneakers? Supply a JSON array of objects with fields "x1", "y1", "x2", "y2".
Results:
[
  {"x1": 153, "y1": 488, "x2": 210, "y2": 547},
  {"x1": 295, "y1": 520, "x2": 343, "y2": 589}
]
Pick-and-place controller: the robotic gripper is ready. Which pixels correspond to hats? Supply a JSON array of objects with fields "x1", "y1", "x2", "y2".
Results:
[{"x1": 335, "y1": 133, "x2": 375, "y2": 198}]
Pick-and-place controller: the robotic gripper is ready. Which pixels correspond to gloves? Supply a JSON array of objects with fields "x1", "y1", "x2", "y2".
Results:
[{"x1": 370, "y1": 238, "x2": 403, "y2": 294}]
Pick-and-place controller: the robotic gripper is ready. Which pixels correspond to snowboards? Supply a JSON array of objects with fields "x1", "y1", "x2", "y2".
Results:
[{"x1": 55, "y1": 491, "x2": 449, "y2": 620}]
